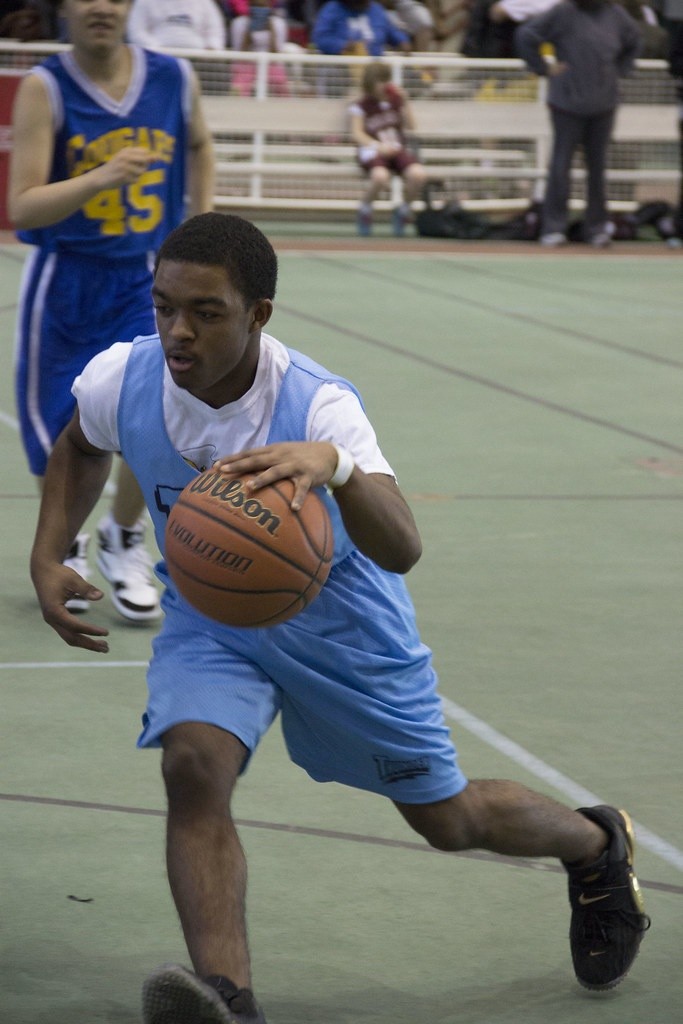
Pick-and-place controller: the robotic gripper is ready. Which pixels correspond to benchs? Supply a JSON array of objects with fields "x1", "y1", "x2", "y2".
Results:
[{"x1": 214, "y1": 144, "x2": 682, "y2": 211}]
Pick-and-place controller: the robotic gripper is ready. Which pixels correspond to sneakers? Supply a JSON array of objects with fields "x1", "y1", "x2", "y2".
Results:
[
  {"x1": 95, "y1": 514, "x2": 163, "y2": 621},
  {"x1": 62, "y1": 534, "x2": 90, "y2": 610},
  {"x1": 142, "y1": 963, "x2": 267, "y2": 1024},
  {"x1": 563, "y1": 804, "x2": 651, "y2": 992}
]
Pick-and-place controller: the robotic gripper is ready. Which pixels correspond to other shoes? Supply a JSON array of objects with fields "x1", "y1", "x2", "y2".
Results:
[
  {"x1": 594, "y1": 235, "x2": 611, "y2": 245},
  {"x1": 541, "y1": 233, "x2": 567, "y2": 245},
  {"x1": 394, "y1": 207, "x2": 408, "y2": 237},
  {"x1": 359, "y1": 211, "x2": 373, "y2": 237}
]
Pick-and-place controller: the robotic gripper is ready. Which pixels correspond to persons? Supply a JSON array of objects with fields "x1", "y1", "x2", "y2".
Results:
[
  {"x1": 6, "y1": 0, "x2": 217, "y2": 624},
  {"x1": 515, "y1": 0, "x2": 640, "y2": 249},
  {"x1": 347, "y1": 63, "x2": 429, "y2": 238},
  {"x1": 27, "y1": 214, "x2": 654, "y2": 1024},
  {"x1": 125, "y1": 0, "x2": 683, "y2": 101}
]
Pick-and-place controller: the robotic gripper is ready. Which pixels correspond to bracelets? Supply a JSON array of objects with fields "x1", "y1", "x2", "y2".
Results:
[{"x1": 326, "y1": 441, "x2": 355, "y2": 490}]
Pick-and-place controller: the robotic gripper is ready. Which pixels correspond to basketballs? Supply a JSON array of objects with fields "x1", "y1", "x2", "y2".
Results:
[{"x1": 164, "y1": 469, "x2": 333, "y2": 627}]
